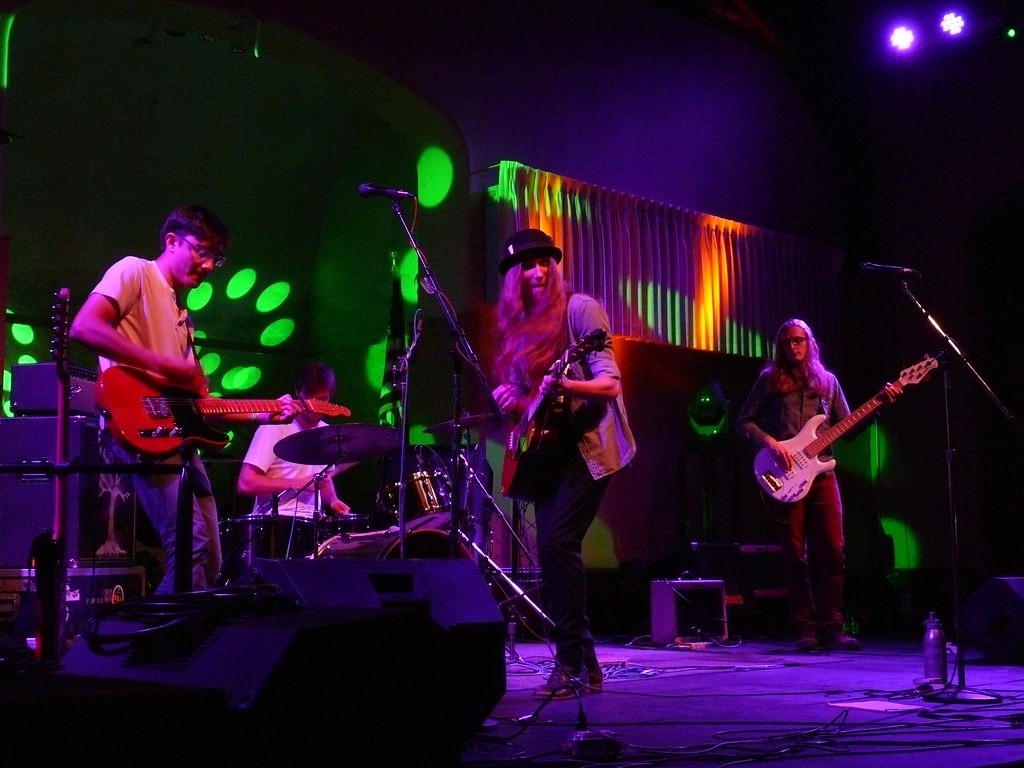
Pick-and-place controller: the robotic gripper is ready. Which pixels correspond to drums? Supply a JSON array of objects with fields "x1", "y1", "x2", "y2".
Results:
[
  {"x1": 307, "y1": 527, "x2": 476, "y2": 561},
  {"x1": 219, "y1": 513, "x2": 317, "y2": 569},
  {"x1": 314, "y1": 513, "x2": 373, "y2": 547},
  {"x1": 374, "y1": 467, "x2": 456, "y2": 531}
]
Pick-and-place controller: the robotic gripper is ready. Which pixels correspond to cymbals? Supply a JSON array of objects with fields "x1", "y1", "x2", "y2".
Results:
[
  {"x1": 273, "y1": 422, "x2": 408, "y2": 467},
  {"x1": 421, "y1": 411, "x2": 512, "y2": 433}
]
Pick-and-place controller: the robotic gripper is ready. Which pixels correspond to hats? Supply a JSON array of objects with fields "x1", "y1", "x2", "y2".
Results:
[{"x1": 498, "y1": 230, "x2": 563, "y2": 274}]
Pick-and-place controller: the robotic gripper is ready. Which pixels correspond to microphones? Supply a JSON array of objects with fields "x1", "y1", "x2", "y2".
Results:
[
  {"x1": 259, "y1": 488, "x2": 290, "y2": 515},
  {"x1": 418, "y1": 445, "x2": 426, "y2": 472},
  {"x1": 415, "y1": 310, "x2": 424, "y2": 335},
  {"x1": 359, "y1": 183, "x2": 415, "y2": 199}
]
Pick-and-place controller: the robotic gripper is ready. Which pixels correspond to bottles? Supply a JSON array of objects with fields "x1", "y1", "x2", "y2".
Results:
[{"x1": 923, "y1": 611, "x2": 948, "y2": 682}]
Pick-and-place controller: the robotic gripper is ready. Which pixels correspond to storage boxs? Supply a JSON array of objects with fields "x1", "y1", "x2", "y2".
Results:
[{"x1": 0, "y1": 568, "x2": 146, "y2": 636}]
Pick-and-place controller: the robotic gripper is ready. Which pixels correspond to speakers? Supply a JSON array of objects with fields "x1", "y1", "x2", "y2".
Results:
[
  {"x1": 955, "y1": 577, "x2": 1024, "y2": 665},
  {"x1": 41, "y1": 556, "x2": 506, "y2": 768},
  {"x1": 651, "y1": 580, "x2": 728, "y2": 645}
]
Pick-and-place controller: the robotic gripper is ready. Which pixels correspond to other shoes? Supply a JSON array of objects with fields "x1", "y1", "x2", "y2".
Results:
[
  {"x1": 826, "y1": 633, "x2": 860, "y2": 650},
  {"x1": 535, "y1": 667, "x2": 588, "y2": 699},
  {"x1": 588, "y1": 667, "x2": 604, "y2": 693},
  {"x1": 802, "y1": 635, "x2": 824, "y2": 652}
]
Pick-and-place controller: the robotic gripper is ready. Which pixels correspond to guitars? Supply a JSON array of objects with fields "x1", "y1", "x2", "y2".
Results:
[
  {"x1": 755, "y1": 357, "x2": 938, "y2": 503},
  {"x1": 93, "y1": 361, "x2": 355, "y2": 459},
  {"x1": 499, "y1": 323, "x2": 611, "y2": 499}
]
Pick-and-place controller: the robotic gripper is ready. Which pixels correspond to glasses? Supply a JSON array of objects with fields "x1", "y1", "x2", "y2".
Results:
[
  {"x1": 182, "y1": 240, "x2": 227, "y2": 269},
  {"x1": 779, "y1": 337, "x2": 808, "y2": 351}
]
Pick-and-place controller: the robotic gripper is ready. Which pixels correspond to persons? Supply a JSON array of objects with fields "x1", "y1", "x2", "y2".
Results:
[
  {"x1": 235, "y1": 363, "x2": 350, "y2": 553},
  {"x1": 738, "y1": 318, "x2": 903, "y2": 651},
  {"x1": 488, "y1": 229, "x2": 637, "y2": 696},
  {"x1": 69, "y1": 206, "x2": 233, "y2": 596}
]
